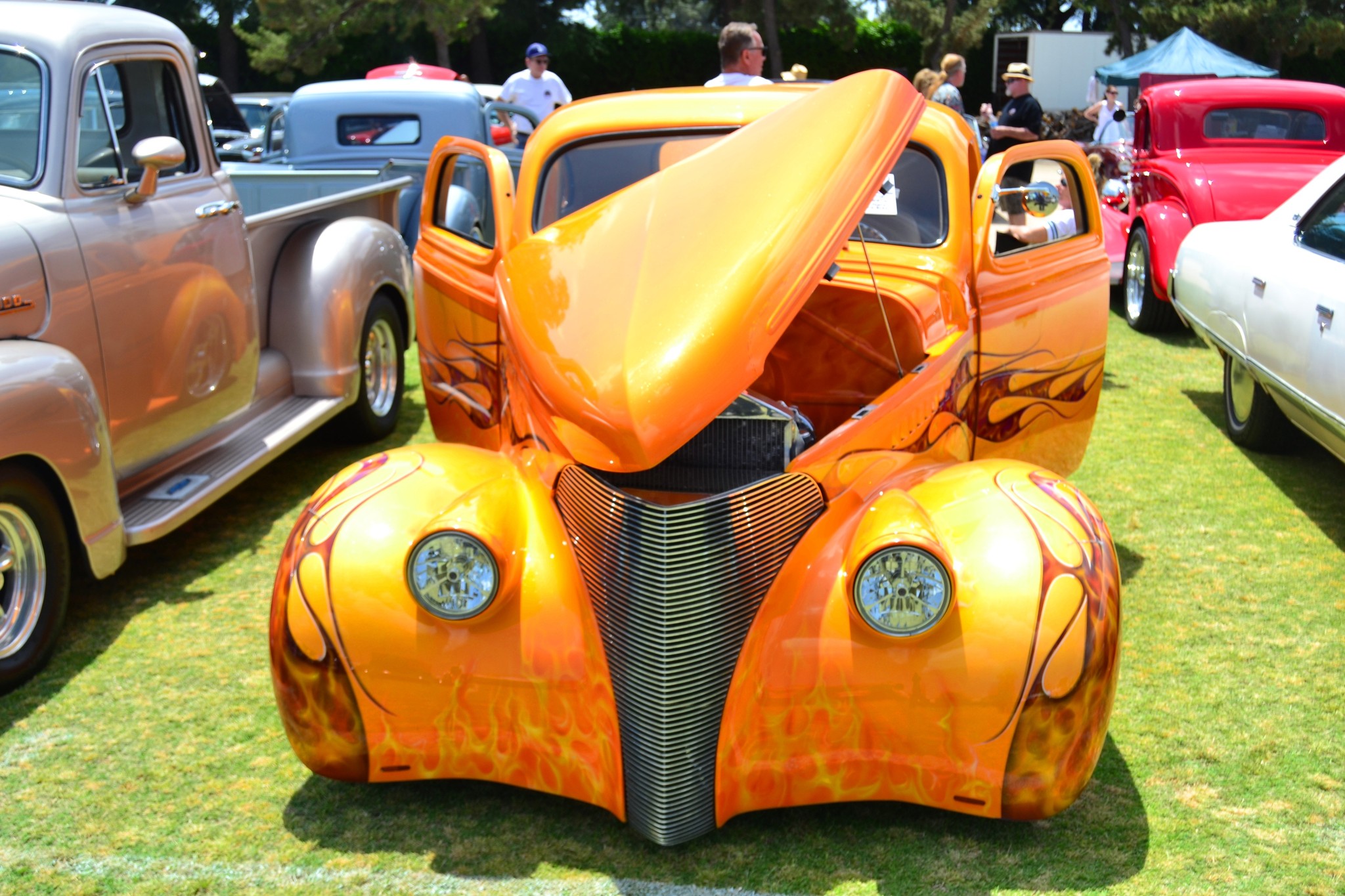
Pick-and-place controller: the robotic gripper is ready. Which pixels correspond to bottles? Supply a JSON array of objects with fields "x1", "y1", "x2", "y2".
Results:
[{"x1": 987, "y1": 114, "x2": 999, "y2": 128}]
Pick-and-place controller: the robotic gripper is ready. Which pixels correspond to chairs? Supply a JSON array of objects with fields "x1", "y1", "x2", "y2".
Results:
[{"x1": 864, "y1": 209, "x2": 921, "y2": 244}]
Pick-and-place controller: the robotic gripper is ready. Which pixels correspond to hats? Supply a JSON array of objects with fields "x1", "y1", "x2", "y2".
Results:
[
  {"x1": 780, "y1": 63, "x2": 808, "y2": 81},
  {"x1": 526, "y1": 43, "x2": 551, "y2": 58},
  {"x1": 913, "y1": 67, "x2": 940, "y2": 97},
  {"x1": 1001, "y1": 62, "x2": 1034, "y2": 82}
]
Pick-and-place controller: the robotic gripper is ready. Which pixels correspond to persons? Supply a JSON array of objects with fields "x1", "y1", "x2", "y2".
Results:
[
  {"x1": 1082, "y1": 84, "x2": 1131, "y2": 144},
  {"x1": 999, "y1": 169, "x2": 1079, "y2": 247},
  {"x1": 495, "y1": 43, "x2": 573, "y2": 148},
  {"x1": 978, "y1": 61, "x2": 1043, "y2": 227},
  {"x1": 913, "y1": 54, "x2": 966, "y2": 119},
  {"x1": 703, "y1": 21, "x2": 778, "y2": 88}
]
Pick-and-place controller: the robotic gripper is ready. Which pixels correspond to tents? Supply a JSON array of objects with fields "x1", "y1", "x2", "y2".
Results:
[{"x1": 1092, "y1": 26, "x2": 1280, "y2": 111}]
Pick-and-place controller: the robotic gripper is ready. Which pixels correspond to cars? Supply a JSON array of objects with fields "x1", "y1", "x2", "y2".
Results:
[
  {"x1": 1090, "y1": 69, "x2": 1345, "y2": 336},
  {"x1": 1, "y1": 0, "x2": 420, "y2": 695},
  {"x1": 1167, "y1": 154, "x2": 1345, "y2": 462},
  {"x1": 273, "y1": 71, "x2": 1129, "y2": 847},
  {"x1": 5, "y1": 64, "x2": 542, "y2": 250}
]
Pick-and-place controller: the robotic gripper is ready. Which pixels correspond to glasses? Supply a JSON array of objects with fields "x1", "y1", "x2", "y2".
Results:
[
  {"x1": 749, "y1": 47, "x2": 768, "y2": 56},
  {"x1": 536, "y1": 60, "x2": 549, "y2": 64},
  {"x1": 1008, "y1": 77, "x2": 1019, "y2": 82},
  {"x1": 1108, "y1": 91, "x2": 1118, "y2": 95}
]
